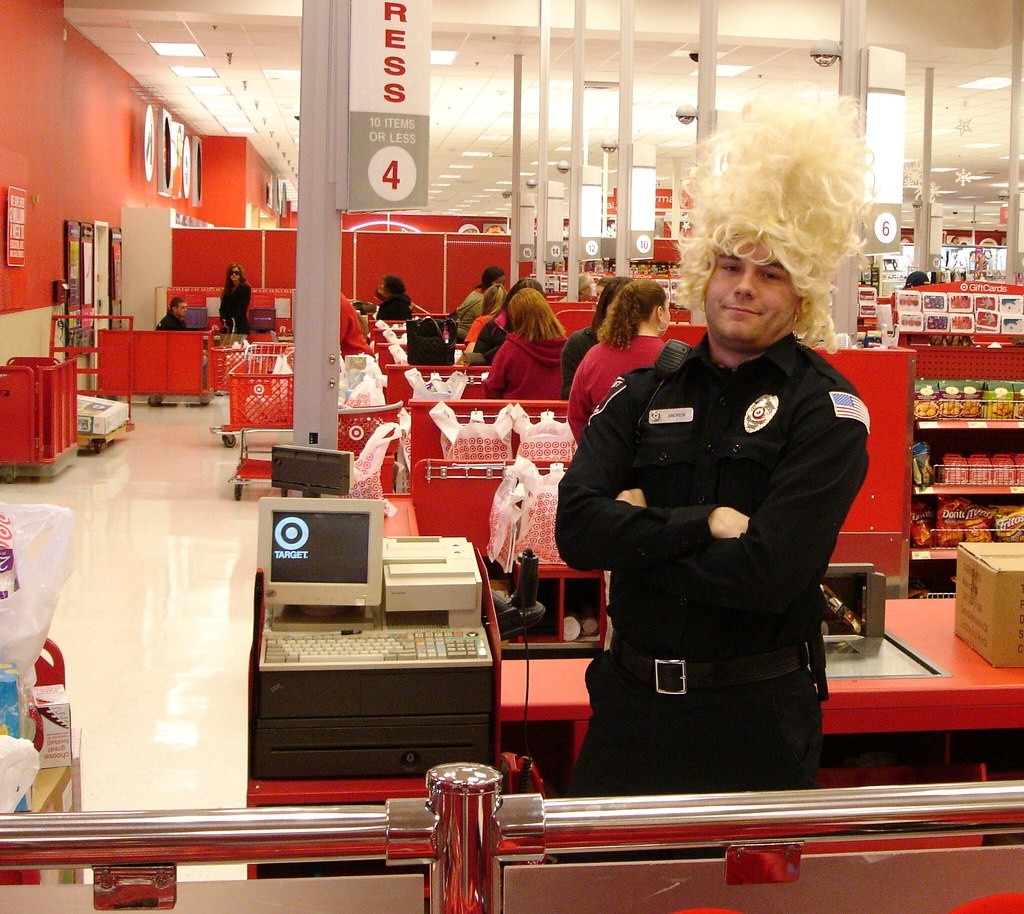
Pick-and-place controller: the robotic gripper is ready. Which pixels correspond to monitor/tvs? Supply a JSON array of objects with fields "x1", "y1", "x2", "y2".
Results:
[
  {"x1": 257, "y1": 495, "x2": 385, "y2": 632},
  {"x1": 248, "y1": 307, "x2": 276, "y2": 334},
  {"x1": 184, "y1": 307, "x2": 207, "y2": 330}
]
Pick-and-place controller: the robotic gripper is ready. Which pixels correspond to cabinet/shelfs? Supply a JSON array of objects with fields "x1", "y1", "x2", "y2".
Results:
[
  {"x1": 859, "y1": 242, "x2": 1024, "y2": 597},
  {"x1": 529, "y1": 261, "x2": 683, "y2": 302}
]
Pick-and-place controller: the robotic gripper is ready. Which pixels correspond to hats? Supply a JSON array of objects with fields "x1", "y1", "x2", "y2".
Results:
[{"x1": 902, "y1": 271, "x2": 929, "y2": 287}]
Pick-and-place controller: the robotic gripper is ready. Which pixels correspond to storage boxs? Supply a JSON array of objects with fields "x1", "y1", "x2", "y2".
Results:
[
  {"x1": 77, "y1": 395, "x2": 129, "y2": 434},
  {"x1": 955, "y1": 541, "x2": 1024, "y2": 667},
  {"x1": 21, "y1": 683, "x2": 73, "y2": 770}
]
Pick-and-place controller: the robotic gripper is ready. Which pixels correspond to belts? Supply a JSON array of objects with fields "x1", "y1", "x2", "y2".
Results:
[{"x1": 610, "y1": 629, "x2": 803, "y2": 695}]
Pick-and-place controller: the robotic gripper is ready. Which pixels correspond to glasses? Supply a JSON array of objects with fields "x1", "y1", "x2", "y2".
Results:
[{"x1": 230, "y1": 271, "x2": 240, "y2": 275}]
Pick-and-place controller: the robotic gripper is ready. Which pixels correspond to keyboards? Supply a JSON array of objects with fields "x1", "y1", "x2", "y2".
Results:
[{"x1": 257, "y1": 623, "x2": 493, "y2": 673}]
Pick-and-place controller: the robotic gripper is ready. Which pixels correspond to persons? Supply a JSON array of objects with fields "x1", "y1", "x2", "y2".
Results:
[
  {"x1": 561, "y1": 276, "x2": 627, "y2": 400},
  {"x1": 464, "y1": 284, "x2": 508, "y2": 353},
  {"x1": 596, "y1": 276, "x2": 614, "y2": 303},
  {"x1": 555, "y1": 97, "x2": 870, "y2": 862},
  {"x1": 949, "y1": 237, "x2": 959, "y2": 245},
  {"x1": 457, "y1": 266, "x2": 506, "y2": 339},
  {"x1": 567, "y1": 279, "x2": 671, "y2": 447},
  {"x1": 474, "y1": 279, "x2": 545, "y2": 364},
  {"x1": 219, "y1": 263, "x2": 251, "y2": 341},
  {"x1": 559, "y1": 274, "x2": 595, "y2": 302},
  {"x1": 283, "y1": 293, "x2": 375, "y2": 363},
  {"x1": 482, "y1": 288, "x2": 568, "y2": 400},
  {"x1": 156, "y1": 297, "x2": 219, "y2": 366},
  {"x1": 377, "y1": 276, "x2": 411, "y2": 319}
]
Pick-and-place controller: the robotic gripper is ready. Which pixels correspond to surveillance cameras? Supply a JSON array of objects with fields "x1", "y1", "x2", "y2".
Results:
[
  {"x1": 601, "y1": 138, "x2": 618, "y2": 154},
  {"x1": 808, "y1": 39, "x2": 843, "y2": 67},
  {"x1": 502, "y1": 190, "x2": 512, "y2": 198},
  {"x1": 525, "y1": 179, "x2": 538, "y2": 189},
  {"x1": 689, "y1": 53, "x2": 698, "y2": 62},
  {"x1": 676, "y1": 104, "x2": 697, "y2": 125},
  {"x1": 556, "y1": 160, "x2": 570, "y2": 174}
]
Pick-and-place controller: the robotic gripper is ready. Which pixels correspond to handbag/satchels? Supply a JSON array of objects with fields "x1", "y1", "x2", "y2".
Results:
[
  {"x1": 406, "y1": 316, "x2": 458, "y2": 366},
  {"x1": 219, "y1": 318, "x2": 247, "y2": 347}
]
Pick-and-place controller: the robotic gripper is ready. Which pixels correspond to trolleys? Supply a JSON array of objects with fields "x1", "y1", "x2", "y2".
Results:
[{"x1": 0, "y1": 314, "x2": 288, "y2": 502}]
